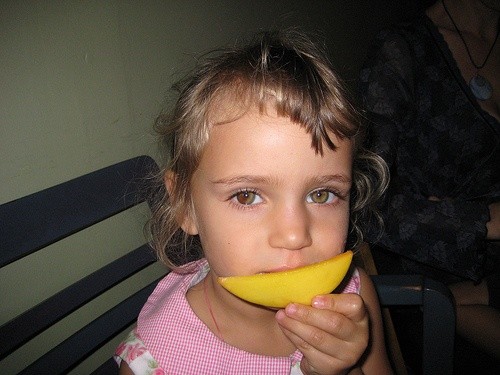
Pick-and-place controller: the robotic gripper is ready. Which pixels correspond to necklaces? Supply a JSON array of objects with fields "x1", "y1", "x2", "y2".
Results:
[{"x1": 440, "y1": 0, "x2": 500, "y2": 100}]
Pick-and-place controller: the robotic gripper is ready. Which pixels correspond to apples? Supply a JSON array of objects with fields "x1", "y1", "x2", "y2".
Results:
[{"x1": 217, "y1": 251, "x2": 354, "y2": 310}]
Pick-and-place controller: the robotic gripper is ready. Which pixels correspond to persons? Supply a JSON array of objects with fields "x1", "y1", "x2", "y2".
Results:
[
  {"x1": 350, "y1": 0, "x2": 500, "y2": 363},
  {"x1": 112, "y1": 27, "x2": 394, "y2": 375}
]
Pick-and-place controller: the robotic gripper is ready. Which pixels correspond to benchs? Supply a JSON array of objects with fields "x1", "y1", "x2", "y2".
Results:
[{"x1": 0, "y1": 155, "x2": 457, "y2": 375}]
